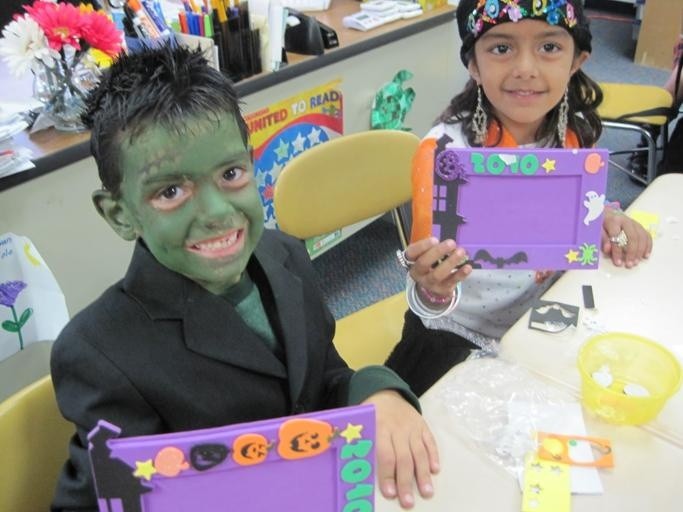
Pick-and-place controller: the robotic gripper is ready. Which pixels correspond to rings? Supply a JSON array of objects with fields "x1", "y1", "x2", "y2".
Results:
[
  {"x1": 609, "y1": 229, "x2": 629, "y2": 246},
  {"x1": 395, "y1": 247, "x2": 416, "y2": 269}
]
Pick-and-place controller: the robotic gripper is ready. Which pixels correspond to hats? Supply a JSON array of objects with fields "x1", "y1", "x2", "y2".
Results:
[{"x1": 456, "y1": 0, "x2": 592, "y2": 68}]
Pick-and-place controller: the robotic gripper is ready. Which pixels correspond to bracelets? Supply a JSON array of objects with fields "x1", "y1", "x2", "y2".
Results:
[{"x1": 420, "y1": 286, "x2": 454, "y2": 305}]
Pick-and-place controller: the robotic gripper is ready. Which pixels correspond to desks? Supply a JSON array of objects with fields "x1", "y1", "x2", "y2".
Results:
[
  {"x1": 4, "y1": 4, "x2": 457, "y2": 377},
  {"x1": 375, "y1": 171, "x2": 683, "y2": 506}
]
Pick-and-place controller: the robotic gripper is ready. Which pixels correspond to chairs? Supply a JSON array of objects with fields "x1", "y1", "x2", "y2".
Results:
[
  {"x1": 0, "y1": 369, "x2": 93, "y2": 509},
  {"x1": 276, "y1": 130, "x2": 423, "y2": 373}
]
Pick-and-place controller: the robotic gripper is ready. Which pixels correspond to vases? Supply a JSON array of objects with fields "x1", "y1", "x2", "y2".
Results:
[{"x1": 28, "y1": 51, "x2": 100, "y2": 132}]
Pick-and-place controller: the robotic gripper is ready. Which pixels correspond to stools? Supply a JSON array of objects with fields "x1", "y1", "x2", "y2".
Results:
[{"x1": 582, "y1": 82, "x2": 672, "y2": 190}]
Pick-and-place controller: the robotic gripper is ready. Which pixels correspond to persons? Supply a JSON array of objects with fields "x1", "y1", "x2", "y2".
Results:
[
  {"x1": 384, "y1": 0, "x2": 654, "y2": 399},
  {"x1": 47, "y1": 35, "x2": 443, "y2": 512}
]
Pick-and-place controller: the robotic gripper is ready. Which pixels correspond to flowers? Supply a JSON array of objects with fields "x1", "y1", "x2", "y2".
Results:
[{"x1": 0, "y1": 1, "x2": 121, "y2": 106}]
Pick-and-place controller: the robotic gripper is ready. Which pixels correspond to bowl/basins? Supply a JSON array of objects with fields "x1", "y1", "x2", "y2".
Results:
[{"x1": 578, "y1": 333, "x2": 682, "y2": 429}]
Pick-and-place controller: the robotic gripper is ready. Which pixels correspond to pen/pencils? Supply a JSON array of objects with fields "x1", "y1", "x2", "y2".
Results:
[
  {"x1": 180, "y1": 0, "x2": 250, "y2": 36},
  {"x1": 122, "y1": 0, "x2": 168, "y2": 36}
]
